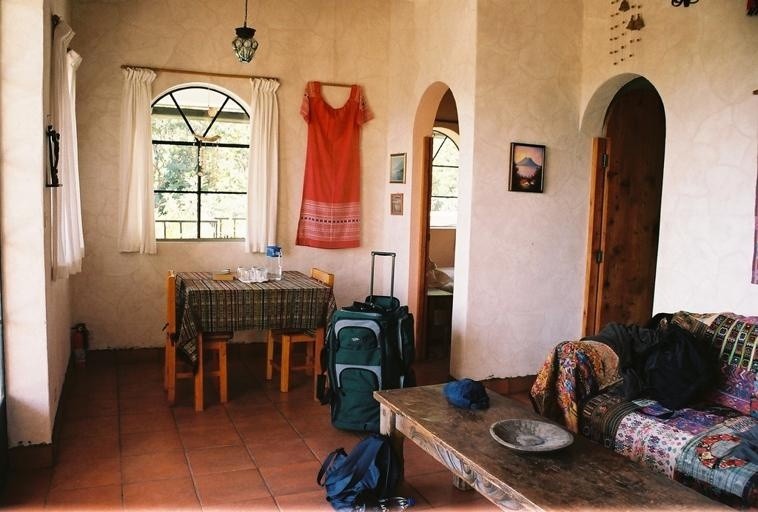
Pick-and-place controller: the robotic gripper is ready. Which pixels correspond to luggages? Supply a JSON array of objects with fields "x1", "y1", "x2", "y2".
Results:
[{"x1": 317, "y1": 252, "x2": 415, "y2": 433}]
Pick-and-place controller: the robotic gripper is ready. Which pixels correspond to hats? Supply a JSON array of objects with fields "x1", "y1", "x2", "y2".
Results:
[{"x1": 444, "y1": 379, "x2": 489, "y2": 410}]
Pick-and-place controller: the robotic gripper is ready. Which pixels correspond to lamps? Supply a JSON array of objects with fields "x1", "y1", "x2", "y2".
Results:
[{"x1": 231, "y1": 1, "x2": 259, "y2": 64}]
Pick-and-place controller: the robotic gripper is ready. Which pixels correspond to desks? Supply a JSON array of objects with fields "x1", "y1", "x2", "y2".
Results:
[
  {"x1": 428, "y1": 288, "x2": 452, "y2": 358},
  {"x1": 175, "y1": 270, "x2": 331, "y2": 413},
  {"x1": 372, "y1": 381, "x2": 741, "y2": 511}
]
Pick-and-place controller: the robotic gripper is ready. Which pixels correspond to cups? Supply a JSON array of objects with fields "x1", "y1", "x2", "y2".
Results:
[{"x1": 236, "y1": 265, "x2": 266, "y2": 283}]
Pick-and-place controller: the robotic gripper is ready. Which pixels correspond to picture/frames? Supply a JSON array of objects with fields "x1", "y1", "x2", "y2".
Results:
[
  {"x1": 508, "y1": 141, "x2": 546, "y2": 192},
  {"x1": 388, "y1": 152, "x2": 407, "y2": 186},
  {"x1": 390, "y1": 192, "x2": 404, "y2": 215}
]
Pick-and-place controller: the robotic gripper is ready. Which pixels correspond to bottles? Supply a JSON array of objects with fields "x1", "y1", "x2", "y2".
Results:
[
  {"x1": 265, "y1": 245, "x2": 283, "y2": 282},
  {"x1": 355, "y1": 496, "x2": 416, "y2": 512}
]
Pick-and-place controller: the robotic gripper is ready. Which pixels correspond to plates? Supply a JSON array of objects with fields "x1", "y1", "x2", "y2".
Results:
[{"x1": 489, "y1": 419, "x2": 577, "y2": 456}]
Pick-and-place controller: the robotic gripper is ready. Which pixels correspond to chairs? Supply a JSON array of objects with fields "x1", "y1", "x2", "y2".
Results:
[
  {"x1": 164, "y1": 269, "x2": 235, "y2": 408},
  {"x1": 265, "y1": 268, "x2": 335, "y2": 394}
]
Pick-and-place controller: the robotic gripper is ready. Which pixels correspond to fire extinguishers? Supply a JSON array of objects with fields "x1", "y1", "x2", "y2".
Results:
[{"x1": 70, "y1": 322, "x2": 89, "y2": 370}]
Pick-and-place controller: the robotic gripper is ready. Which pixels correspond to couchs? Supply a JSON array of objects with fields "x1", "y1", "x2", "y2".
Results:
[{"x1": 532, "y1": 311, "x2": 758, "y2": 512}]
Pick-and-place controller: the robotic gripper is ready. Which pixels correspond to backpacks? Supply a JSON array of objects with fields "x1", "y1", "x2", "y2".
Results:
[
  {"x1": 635, "y1": 312, "x2": 720, "y2": 418},
  {"x1": 317, "y1": 435, "x2": 403, "y2": 510}
]
